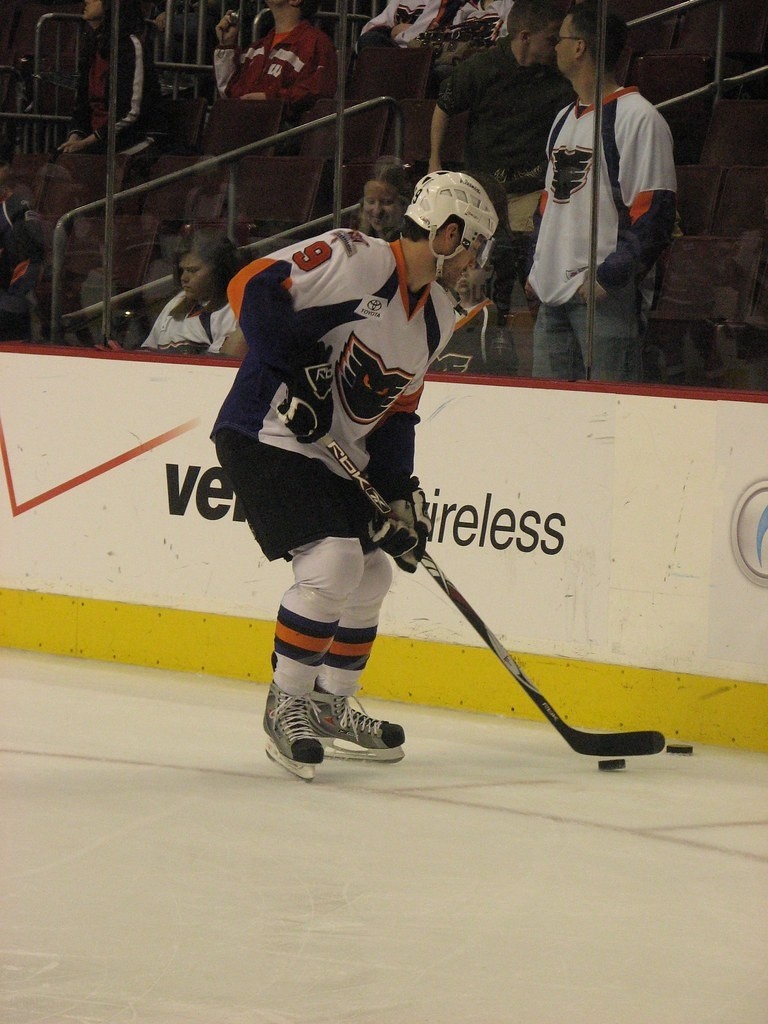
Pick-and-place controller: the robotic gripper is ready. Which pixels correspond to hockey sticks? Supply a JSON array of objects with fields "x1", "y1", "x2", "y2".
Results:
[{"x1": 276, "y1": 396, "x2": 666, "y2": 756}]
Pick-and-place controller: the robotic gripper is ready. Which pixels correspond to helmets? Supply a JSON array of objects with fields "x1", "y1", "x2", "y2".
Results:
[{"x1": 404, "y1": 170, "x2": 499, "y2": 269}]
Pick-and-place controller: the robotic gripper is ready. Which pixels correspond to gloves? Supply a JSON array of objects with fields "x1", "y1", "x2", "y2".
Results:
[
  {"x1": 358, "y1": 476, "x2": 433, "y2": 573},
  {"x1": 276, "y1": 341, "x2": 334, "y2": 444}
]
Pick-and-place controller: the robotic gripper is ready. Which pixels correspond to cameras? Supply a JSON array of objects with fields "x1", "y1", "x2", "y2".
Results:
[{"x1": 229, "y1": 9, "x2": 241, "y2": 26}]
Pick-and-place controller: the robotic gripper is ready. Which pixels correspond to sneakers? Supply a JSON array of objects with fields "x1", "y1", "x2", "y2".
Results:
[
  {"x1": 262, "y1": 651, "x2": 324, "y2": 778},
  {"x1": 308, "y1": 690, "x2": 405, "y2": 764}
]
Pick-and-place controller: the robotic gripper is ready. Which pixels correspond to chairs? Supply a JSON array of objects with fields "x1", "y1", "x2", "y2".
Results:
[{"x1": 0, "y1": 0, "x2": 768, "y2": 393}]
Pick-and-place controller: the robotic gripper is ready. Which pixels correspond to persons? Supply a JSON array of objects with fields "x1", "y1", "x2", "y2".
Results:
[
  {"x1": 209, "y1": 170, "x2": 499, "y2": 763},
  {"x1": 0, "y1": 0, "x2": 679, "y2": 383}
]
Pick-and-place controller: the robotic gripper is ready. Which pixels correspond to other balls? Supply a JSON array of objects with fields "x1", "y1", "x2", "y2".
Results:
[
  {"x1": 598, "y1": 759, "x2": 626, "y2": 770},
  {"x1": 666, "y1": 744, "x2": 693, "y2": 755}
]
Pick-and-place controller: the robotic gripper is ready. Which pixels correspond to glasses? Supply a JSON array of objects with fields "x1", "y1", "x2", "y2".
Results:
[{"x1": 550, "y1": 33, "x2": 593, "y2": 46}]
